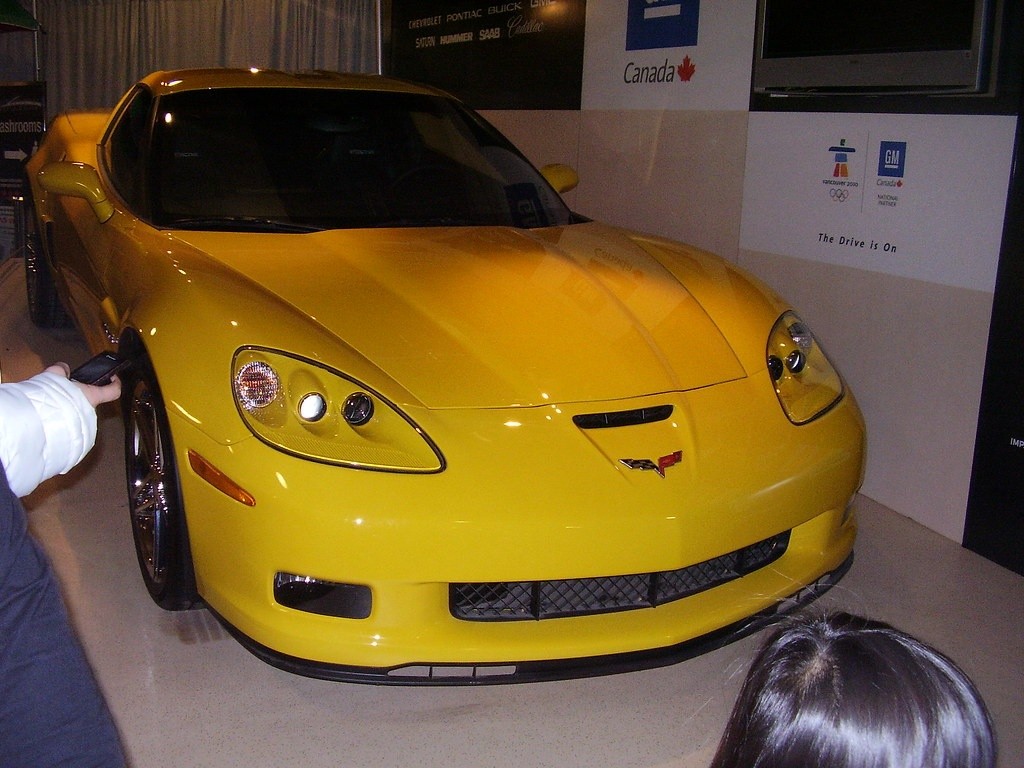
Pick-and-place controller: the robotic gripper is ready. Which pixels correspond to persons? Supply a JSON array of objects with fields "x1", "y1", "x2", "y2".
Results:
[
  {"x1": 706, "y1": 608, "x2": 1002, "y2": 768},
  {"x1": 1, "y1": 361, "x2": 127, "y2": 768}
]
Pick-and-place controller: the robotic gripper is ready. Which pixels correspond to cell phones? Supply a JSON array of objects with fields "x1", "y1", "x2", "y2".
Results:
[{"x1": 69, "y1": 349, "x2": 132, "y2": 386}]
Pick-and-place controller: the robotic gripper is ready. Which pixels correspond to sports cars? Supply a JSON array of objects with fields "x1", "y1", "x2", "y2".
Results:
[{"x1": 20, "y1": 64, "x2": 869, "y2": 689}]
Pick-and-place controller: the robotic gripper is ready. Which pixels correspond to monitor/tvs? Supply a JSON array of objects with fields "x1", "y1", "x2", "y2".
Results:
[{"x1": 754, "y1": 0, "x2": 996, "y2": 95}]
